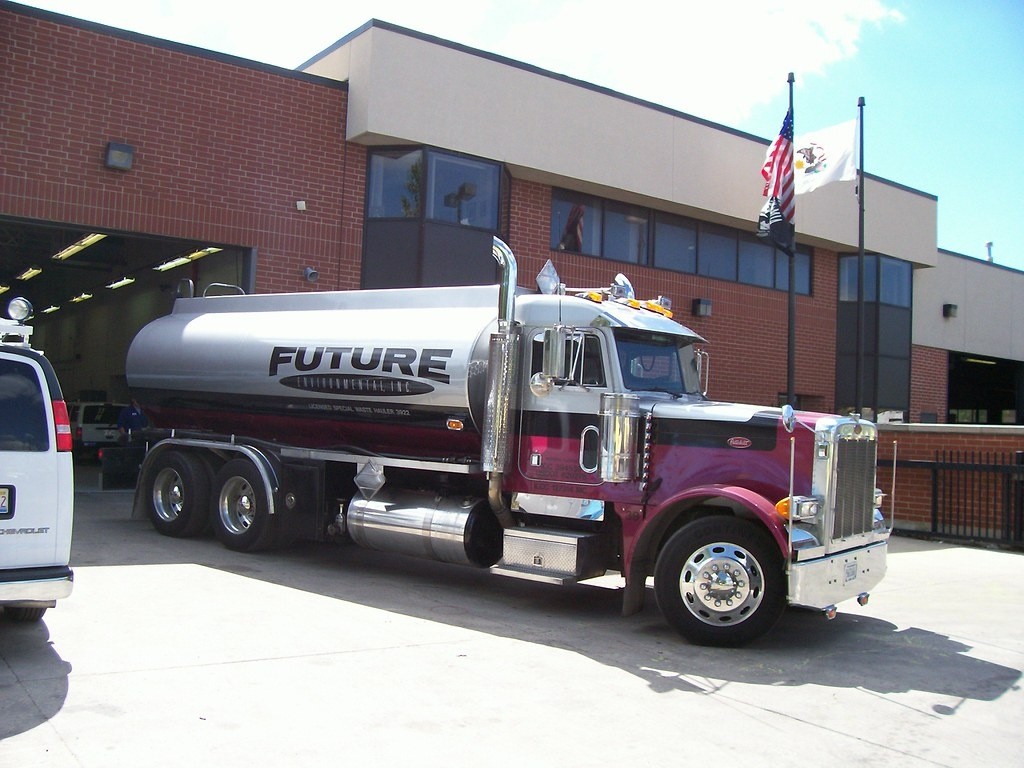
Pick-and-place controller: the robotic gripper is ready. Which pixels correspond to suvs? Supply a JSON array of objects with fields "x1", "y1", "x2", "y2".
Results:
[{"x1": 1, "y1": 295, "x2": 75, "y2": 621}]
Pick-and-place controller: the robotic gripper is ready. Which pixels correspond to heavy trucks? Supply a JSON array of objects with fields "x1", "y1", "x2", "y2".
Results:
[{"x1": 123, "y1": 236, "x2": 899, "y2": 649}]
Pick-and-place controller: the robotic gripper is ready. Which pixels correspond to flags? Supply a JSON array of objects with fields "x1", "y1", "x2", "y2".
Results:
[
  {"x1": 757, "y1": 107, "x2": 796, "y2": 259},
  {"x1": 793, "y1": 118, "x2": 858, "y2": 195},
  {"x1": 560, "y1": 203, "x2": 586, "y2": 253}
]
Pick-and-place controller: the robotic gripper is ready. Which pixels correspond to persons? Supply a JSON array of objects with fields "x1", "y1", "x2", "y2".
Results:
[{"x1": 117, "y1": 397, "x2": 149, "y2": 435}]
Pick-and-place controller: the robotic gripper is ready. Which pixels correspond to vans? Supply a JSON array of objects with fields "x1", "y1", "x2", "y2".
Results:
[{"x1": 66, "y1": 401, "x2": 132, "y2": 466}]
